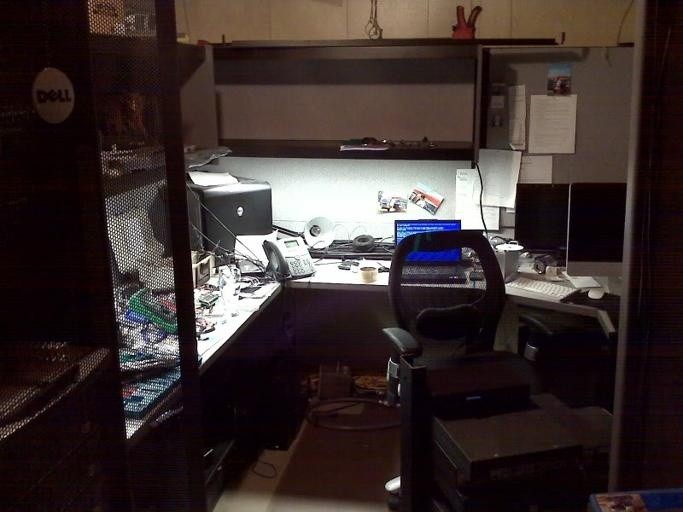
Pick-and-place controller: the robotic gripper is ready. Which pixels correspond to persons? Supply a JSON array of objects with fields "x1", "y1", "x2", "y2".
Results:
[{"x1": 413, "y1": 193, "x2": 426, "y2": 208}]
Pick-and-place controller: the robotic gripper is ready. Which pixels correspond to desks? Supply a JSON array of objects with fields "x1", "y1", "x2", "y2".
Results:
[{"x1": 113, "y1": 233, "x2": 616, "y2": 511}]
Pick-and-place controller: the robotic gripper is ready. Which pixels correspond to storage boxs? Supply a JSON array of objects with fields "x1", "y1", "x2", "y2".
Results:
[{"x1": 87, "y1": 0, "x2": 125, "y2": 37}]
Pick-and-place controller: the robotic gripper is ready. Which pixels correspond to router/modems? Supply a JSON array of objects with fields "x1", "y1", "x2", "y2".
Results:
[{"x1": 495, "y1": 251, "x2": 519, "y2": 283}]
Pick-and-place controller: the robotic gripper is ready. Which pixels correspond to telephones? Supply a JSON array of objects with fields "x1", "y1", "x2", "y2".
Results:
[{"x1": 262, "y1": 238, "x2": 316, "y2": 282}]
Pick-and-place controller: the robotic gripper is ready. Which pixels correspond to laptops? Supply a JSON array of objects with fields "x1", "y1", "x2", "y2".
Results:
[{"x1": 394, "y1": 219, "x2": 466, "y2": 283}]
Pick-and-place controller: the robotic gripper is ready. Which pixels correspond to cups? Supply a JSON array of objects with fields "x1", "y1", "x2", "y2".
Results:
[{"x1": 219, "y1": 265, "x2": 240, "y2": 319}]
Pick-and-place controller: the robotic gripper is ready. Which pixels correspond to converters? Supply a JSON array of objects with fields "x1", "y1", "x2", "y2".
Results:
[{"x1": 470, "y1": 271, "x2": 483, "y2": 281}]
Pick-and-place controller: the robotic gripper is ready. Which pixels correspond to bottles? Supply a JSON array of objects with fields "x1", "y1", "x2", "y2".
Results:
[
  {"x1": 547, "y1": 65, "x2": 572, "y2": 96},
  {"x1": 350, "y1": 262, "x2": 359, "y2": 273}
]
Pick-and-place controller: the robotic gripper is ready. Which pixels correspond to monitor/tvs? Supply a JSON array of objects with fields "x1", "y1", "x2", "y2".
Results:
[
  {"x1": 567, "y1": 182, "x2": 627, "y2": 276},
  {"x1": 513, "y1": 183, "x2": 571, "y2": 266}
]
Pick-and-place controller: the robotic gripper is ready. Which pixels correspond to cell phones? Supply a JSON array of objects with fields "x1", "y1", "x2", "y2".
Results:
[{"x1": 339, "y1": 261, "x2": 359, "y2": 270}]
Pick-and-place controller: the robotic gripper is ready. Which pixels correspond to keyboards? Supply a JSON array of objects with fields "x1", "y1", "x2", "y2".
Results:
[{"x1": 507, "y1": 277, "x2": 578, "y2": 300}]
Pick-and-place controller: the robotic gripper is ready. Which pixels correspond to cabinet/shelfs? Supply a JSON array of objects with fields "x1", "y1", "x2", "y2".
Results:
[
  {"x1": 90, "y1": 31, "x2": 219, "y2": 200},
  {"x1": 0, "y1": 347, "x2": 127, "y2": 511}
]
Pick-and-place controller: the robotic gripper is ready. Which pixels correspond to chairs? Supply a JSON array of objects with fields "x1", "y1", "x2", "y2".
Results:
[{"x1": 381, "y1": 229, "x2": 564, "y2": 420}]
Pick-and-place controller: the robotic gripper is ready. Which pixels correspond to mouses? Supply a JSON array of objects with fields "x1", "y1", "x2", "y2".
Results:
[{"x1": 588, "y1": 287, "x2": 605, "y2": 300}]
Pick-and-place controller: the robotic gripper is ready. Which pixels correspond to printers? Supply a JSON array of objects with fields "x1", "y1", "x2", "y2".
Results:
[{"x1": 187, "y1": 172, "x2": 272, "y2": 269}]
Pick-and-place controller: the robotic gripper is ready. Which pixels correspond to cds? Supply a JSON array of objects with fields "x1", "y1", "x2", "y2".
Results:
[{"x1": 495, "y1": 243, "x2": 524, "y2": 251}]
